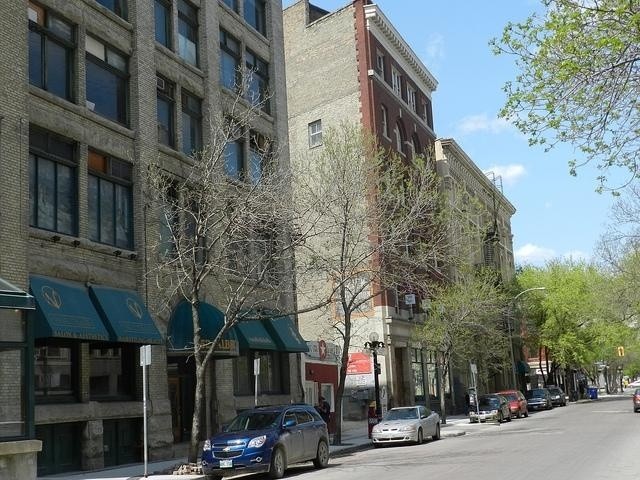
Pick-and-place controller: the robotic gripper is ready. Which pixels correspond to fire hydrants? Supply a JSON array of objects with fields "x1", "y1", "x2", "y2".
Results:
[{"x1": 368, "y1": 406, "x2": 378, "y2": 439}]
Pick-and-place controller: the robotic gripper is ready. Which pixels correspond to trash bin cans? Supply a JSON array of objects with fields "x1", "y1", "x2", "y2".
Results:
[{"x1": 588, "y1": 388, "x2": 597, "y2": 399}]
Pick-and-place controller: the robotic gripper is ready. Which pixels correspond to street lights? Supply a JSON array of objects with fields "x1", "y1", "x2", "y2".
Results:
[
  {"x1": 362, "y1": 332, "x2": 388, "y2": 424},
  {"x1": 507, "y1": 287, "x2": 546, "y2": 389}
]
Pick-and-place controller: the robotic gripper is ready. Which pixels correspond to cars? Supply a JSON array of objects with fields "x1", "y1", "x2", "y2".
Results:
[
  {"x1": 633, "y1": 389, "x2": 640, "y2": 412},
  {"x1": 371, "y1": 405, "x2": 440, "y2": 448},
  {"x1": 469, "y1": 386, "x2": 568, "y2": 423},
  {"x1": 202, "y1": 402, "x2": 330, "y2": 480},
  {"x1": 628, "y1": 381, "x2": 640, "y2": 388}
]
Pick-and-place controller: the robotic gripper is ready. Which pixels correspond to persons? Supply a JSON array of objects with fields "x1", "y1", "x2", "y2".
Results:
[
  {"x1": 524, "y1": 386, "x2": 528, "y2": 395},
  {"x1": 579, "y1": 381, "x2": 585, "y2": 399},
  {"x1": 463, "y1": 392, "x2": 473, "y2": 416},
  {"x1": 317, "y1": 395, "x2": 330, "y2": 442},
  {"x1": 624, "y1": 380, "x2": 628, "y2": 389}
]
